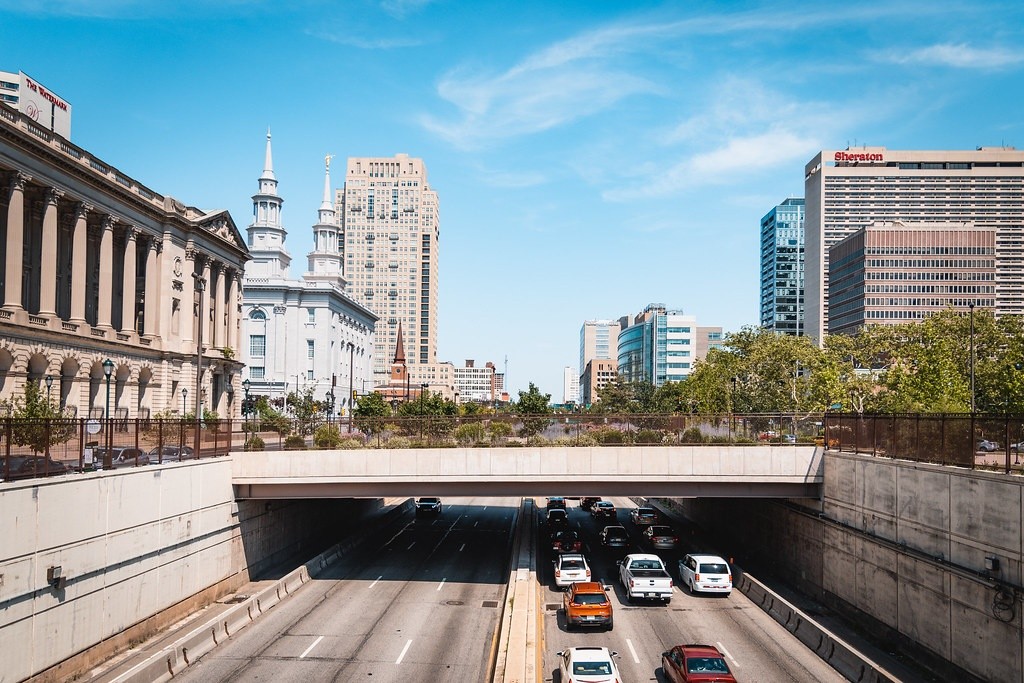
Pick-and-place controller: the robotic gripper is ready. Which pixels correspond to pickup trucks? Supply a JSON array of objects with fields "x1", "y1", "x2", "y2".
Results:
[{"x1": 616, "y1": 553, "x2": 674, "y2": 603}]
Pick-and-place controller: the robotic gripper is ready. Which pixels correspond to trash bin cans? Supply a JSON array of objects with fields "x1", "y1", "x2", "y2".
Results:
[{"x1": 85, "y1": 441, "x2": 98, "y2": 463}]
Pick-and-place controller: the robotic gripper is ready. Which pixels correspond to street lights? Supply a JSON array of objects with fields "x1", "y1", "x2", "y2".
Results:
[
  {"x1": 102, "y1": 359, "x2": 114, "y2": 454},
  {"x1": 244, "y1": 378, "x2": 252, "y2": 445},
  {"x1": 45, "y1": 374, "x2": 54, "y2": 413},
  {"x1": 421, "y1": 382, "x2": 429, "y2": 441},
  {"x1": 182, "y1": 387, "x2": 188, "y2": 446},
  {"x1": 325, "y1": 391, "x2": 332, "y2": 423}
]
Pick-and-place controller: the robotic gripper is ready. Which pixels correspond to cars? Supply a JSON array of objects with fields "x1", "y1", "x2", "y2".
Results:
[
  {"x1": 770, "y1": 434, "x2": 796, "y2": 446},
  {"x1": 147, "y1": 446, "x2": 194, "y2": 465},
  {"x1": 556, "y1": 646, "x2": 624, "y2": 683},
  {"x1": 678, "y1": 553, "x2": 733, "y2": 596},
  {"x1": 976, "y1": 438, "x2": 999, "y2": 452},
  {"x1": 642, "y1": 525, "x2": 680, "y2": 550},
  {"x1": 546, "y1": 496, "x2": 631, "y2": 556},
  {"x1": 551, "y1": 553, "x2": 591, "y2": 588},
  {"x1": 662, "y1": 645, "x2": 738, "y2": 683},
  {"x1": 1011, "y1": 440, "x2": 1024, "y2": 453},
  {"x1": 67, "y1": 446, "x2": 149, "y2": 472},
  {"x1": 631, "y1": 508, "x2": 657, "y2": 527},
  {"x1": 415, "y1": 496, "x2": 442, "y2": 516},
  {"x1": 0, "y1": 454, "x2": 71, "y2": 483}
]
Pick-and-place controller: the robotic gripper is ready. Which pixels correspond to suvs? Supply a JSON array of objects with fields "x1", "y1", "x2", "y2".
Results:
[{"x1": 562, "y1": 581, "x2": 614, "y2": 631}]
